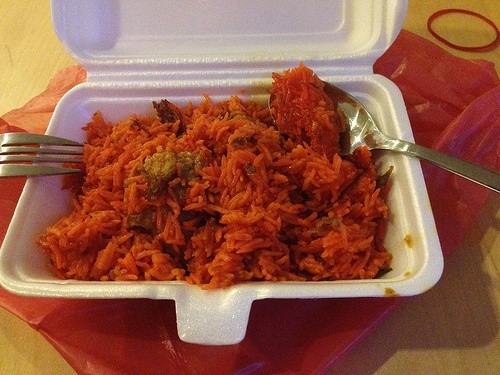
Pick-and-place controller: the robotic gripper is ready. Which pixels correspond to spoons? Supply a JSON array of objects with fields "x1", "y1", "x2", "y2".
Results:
[{"x1": 266, "y1": 80, "x2": 500, "y2": 196}]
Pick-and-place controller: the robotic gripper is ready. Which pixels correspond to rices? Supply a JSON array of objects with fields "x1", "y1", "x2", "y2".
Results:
[{"x1": 33, "y1": 62, "x2": 395, "y2": 290}]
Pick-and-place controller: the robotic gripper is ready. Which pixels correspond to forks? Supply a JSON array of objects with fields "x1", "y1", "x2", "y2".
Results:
[{"x1": 0, "y1": 131, "x2": 86, "y2": 179}]
